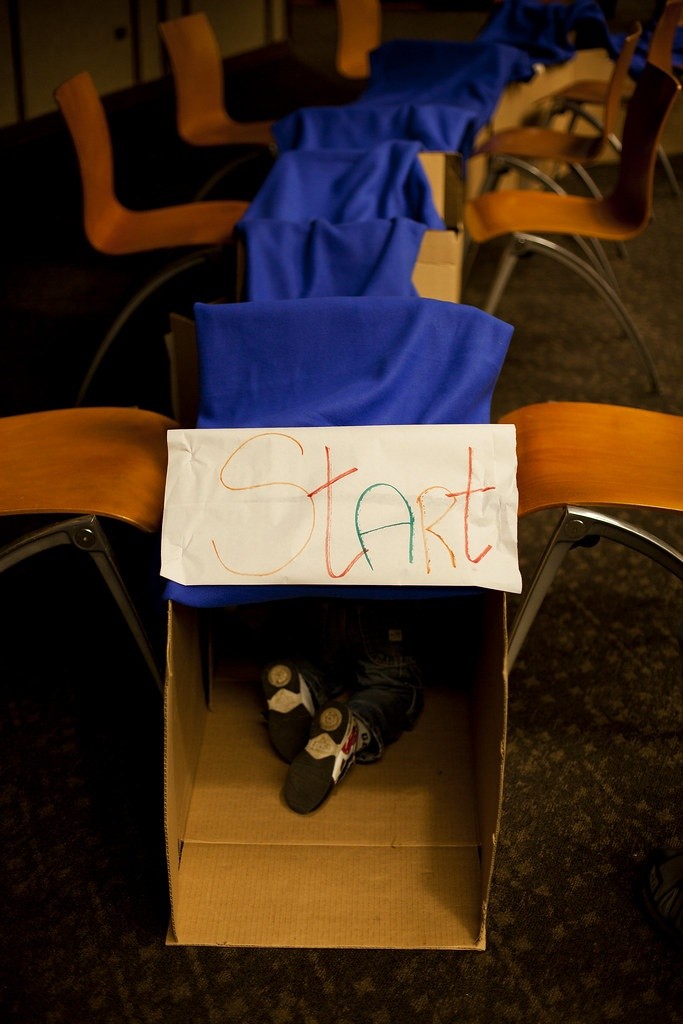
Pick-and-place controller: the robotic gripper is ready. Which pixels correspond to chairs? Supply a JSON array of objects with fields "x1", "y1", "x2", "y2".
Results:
[
  {"x1": 265, "y1": 137, "x2": 459, "y2": 232},
  {"x1": 57, "y1": 72, "x2": 253, "y2": 390},
  {"x1": 334, "y1": 0, "x2": 384, "y2": 87},
  {"x1": 181, "y1": 295, "x2": 509, "y2": 430},
  {"x1": 161, "y1": 14, "x2": 281, "y2": 201},
  {"x1": 450, "y1": 61, "x2": 680, "y2": 388},
  {"x1": 504, "y1": 402, "x2": 683, "y2": 684},
  {"x1": 0, "y1": 405, "x2": 170, "y2": 694},
  {"x1": 245, "y1": 224, "x2": 470, "y2": 300},
  {"x1": 535, "y1": 0, "x2": 682, "y2": 165},
  {"x1": 467, "y1": 27, "x2": 644, "y2": 209}
]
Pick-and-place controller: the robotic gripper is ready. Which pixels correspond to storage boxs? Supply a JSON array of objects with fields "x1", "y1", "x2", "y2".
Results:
[
  {"x1": 154, "y1": 419, "x2": 522, "y2": 960},
  {"x1": 274, "y1": 10, "x2": 598, "y2": 142}
]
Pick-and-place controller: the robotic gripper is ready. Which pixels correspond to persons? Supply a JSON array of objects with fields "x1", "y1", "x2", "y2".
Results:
[{"x1": 258, "y1": 597, "x2": 423, "y2": 814}]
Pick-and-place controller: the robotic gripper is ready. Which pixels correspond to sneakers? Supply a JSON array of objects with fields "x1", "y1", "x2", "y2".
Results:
[
  {"x1": 285, "y1": 701, "x2": 357, "y2": 814},
  {"x1": 261, "y1": 661, "x2": 314, "y2": 762}
]
